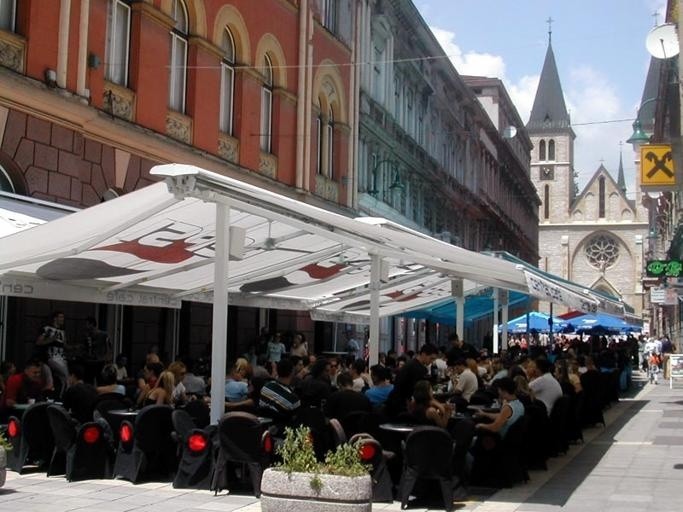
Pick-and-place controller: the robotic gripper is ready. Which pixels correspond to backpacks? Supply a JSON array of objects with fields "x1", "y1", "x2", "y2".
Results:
[{"x1": 641, "y1": 349, "x2": 652, "y2": 360}]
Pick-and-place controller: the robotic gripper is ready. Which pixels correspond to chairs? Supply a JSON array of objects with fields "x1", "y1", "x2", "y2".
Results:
[{"x1": 0, "y1": 340, "x2": 637, "y2": 512}]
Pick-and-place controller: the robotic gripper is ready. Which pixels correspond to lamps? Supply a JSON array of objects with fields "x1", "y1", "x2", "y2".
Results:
[
  {"x1": 625, "y1": 97, "x2": 674, "y2": 145},
  {"x1": 367, "y1": 158, "x2": 407, "y2": 197},
  {"x1": 646, "y1": 213, "x2": 671, "y2": 240}
]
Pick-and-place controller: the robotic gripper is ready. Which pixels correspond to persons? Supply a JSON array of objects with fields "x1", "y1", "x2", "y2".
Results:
[
  {"x1": 224, "y1": 326, "x2": 536, "y2": 490},
  {"x1": 507, "y1": 331, "x2": 671, "y2": 420},
  {"x1": 2, "y1": 310, "x2": 212, "y2": 465}
]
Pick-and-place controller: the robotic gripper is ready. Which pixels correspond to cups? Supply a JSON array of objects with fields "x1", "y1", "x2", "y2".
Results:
[{"x1": 27, "y1": 398, "x2": 35, "y2": 406}]
